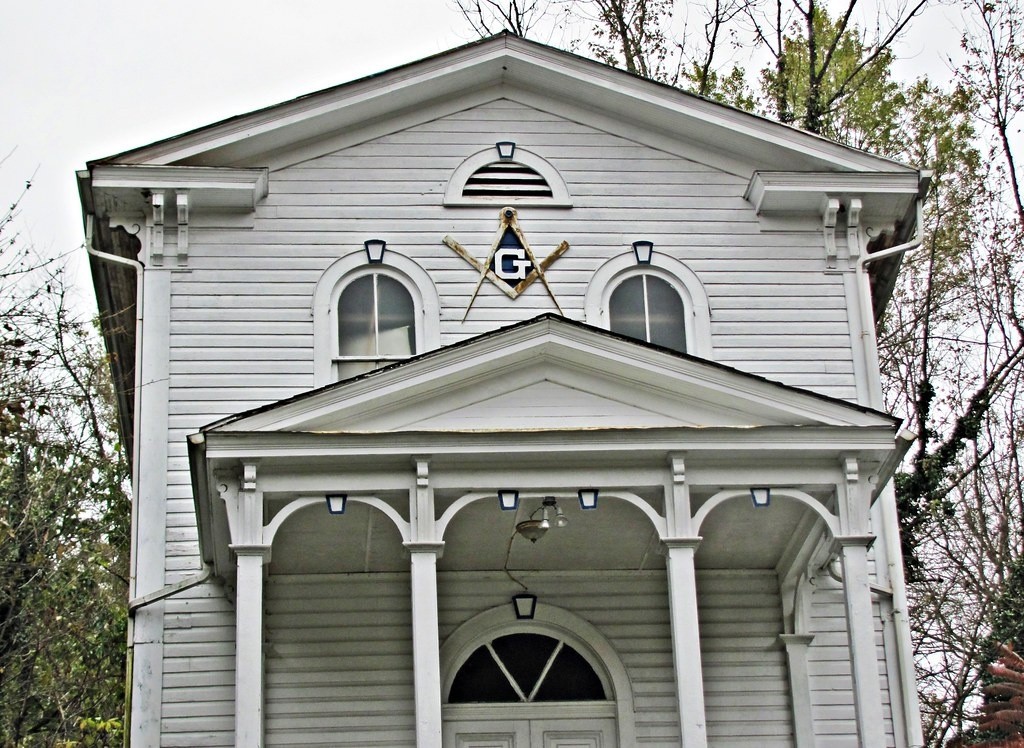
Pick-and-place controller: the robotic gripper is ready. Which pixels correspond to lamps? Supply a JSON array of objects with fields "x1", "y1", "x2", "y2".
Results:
[{"x1": 514, "y1": 506, "x2": 570, "y2": 543}]
